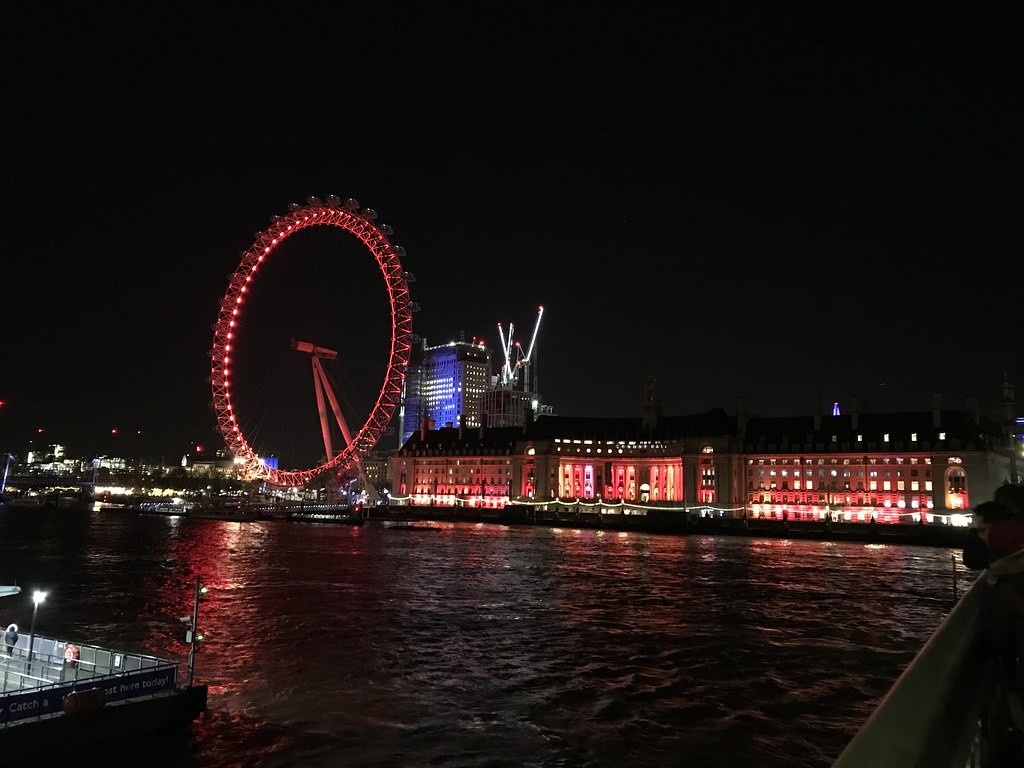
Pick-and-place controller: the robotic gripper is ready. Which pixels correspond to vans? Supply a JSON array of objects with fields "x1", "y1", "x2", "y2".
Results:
[{"x1": 498, "y1": 505, "x2": 534, "y2": 521}]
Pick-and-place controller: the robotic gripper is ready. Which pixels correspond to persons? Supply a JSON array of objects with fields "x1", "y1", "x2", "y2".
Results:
[
  {"x1": 4, "y1": 627, "x2": 18, "y2": 657},
  {"x1": 963, "y1": 485, "x2": 1024, "y2": 708}
]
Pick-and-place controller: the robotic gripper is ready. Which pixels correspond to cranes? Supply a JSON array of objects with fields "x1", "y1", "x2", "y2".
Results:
[
  {"x1": 517, "y1": 307, "x2": 543, "y2": 392},
  {"x1": 498, "y1": 323, "x2": 517, "y2": 383},
  {"x1": 501, "y1": 323, "x2": 513, "y2": 384}
]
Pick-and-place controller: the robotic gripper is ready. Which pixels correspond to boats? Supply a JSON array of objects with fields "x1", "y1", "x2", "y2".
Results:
[{"x1": 189, "y1": 497, "x2": 258, "y2": 520}]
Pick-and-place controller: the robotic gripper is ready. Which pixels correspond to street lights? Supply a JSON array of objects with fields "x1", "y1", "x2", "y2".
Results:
[
  {"x1": 188, "y1": 575, "x2": 208, "y2": 685},
  {"x1": 27, "y1": 591, "x2": 43, "y2": 675}
]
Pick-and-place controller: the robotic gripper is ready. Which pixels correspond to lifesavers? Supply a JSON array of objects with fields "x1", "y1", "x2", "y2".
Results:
[{"x1": 64, "y1": 644, "x2": 79, "y2": 665}]
